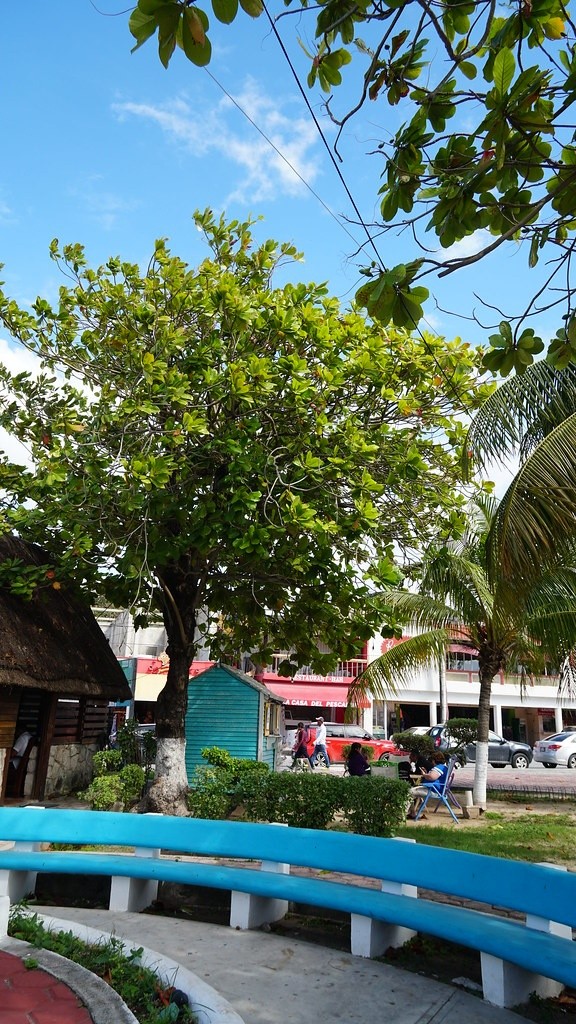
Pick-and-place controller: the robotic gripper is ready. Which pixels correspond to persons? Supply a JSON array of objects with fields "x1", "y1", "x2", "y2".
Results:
[
  {"x1": 287, "y1": 722, "x2": 315, "y2": 770},
  {"x1": 407, "y1": 750, "x2": 448, "y2": 819},
  {"x1": 5, "y1": 724, "x2": 32, "y2": 798},
  {"x1": 311, "y1": 716, "x2": 330, "y2": 768},
  {"x1": 347, "y1": 742, "x2": 371, "y2": 776},
  {"x1": 143, "y1": 710, "x2": 152, "y2": 723},
  {"x1": 409, "y1": 751, "x2": 434, "y2": 783}
]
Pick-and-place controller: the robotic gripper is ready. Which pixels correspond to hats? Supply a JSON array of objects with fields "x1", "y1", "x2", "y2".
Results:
[{"x1": 315, "y1": 717, "x2": 324, "y2": 722}]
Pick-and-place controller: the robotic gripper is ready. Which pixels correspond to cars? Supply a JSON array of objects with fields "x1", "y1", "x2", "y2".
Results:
[
  {"x1": 292, "y1": 721, "x2": 411, "y2": 768},
  {"x1": 389, "y1": 726, "x2": 432, "y2": 748},
  {"x1": 428, "y1": 722, "x2": 533, "y2": 770},
  {"x1": 532, "y1": 731, "x2": 576, "y2": 769}
]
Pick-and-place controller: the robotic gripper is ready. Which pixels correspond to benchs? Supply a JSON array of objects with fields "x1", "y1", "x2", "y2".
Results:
[{"x1": 0, "y1": 806, "x2": 575, "y2": 1008}]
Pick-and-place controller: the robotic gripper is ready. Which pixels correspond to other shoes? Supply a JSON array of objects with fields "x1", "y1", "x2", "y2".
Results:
[
  {"x1": 407, "y1": 813, "x2": 417, "y2": 819},
  {"x1": 287, "y1": 766, "x2": 293, "y2": 769}
]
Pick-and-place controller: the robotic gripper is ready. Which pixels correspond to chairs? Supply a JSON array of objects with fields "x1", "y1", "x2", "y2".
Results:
[
  {"x1": 370, "y1": 761, "x2": 399, "y2": 780},
  {"x1": 415, "y1": 757, "x2": 462, "y2": 825},
  {"x1": 6, "y1": 736, "x2": 37, "y2": 797}
]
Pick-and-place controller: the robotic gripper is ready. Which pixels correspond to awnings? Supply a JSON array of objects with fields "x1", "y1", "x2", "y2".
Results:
[{"x1": 265, "y1": 674, "x2": 370, "y2": 708}]
